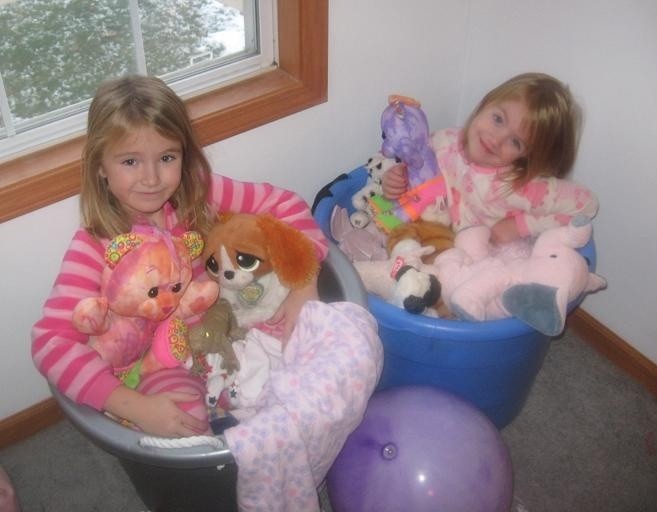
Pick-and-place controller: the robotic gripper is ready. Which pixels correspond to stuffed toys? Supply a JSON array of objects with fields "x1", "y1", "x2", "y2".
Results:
[
  {"x1": 202, "y1": 212, "x2": 321, "y2": 333},
  {"x1": 330, "y1": 92, "x2": 608, "y2": 337},
  {"x1": 331, "y1": 95, "x2": 607, "y2": 336},
  {"x1": 72, "y1": 227, "x2": 240, "y2": 373}
]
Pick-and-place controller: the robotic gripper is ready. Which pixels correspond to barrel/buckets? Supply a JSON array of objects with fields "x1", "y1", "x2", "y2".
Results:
[
  {"x1": 42, "y1": 240, "x2": 366, "y2": 512},
  {"x1": 312, "y1": 164, "x2": 597, "y2": 433}
]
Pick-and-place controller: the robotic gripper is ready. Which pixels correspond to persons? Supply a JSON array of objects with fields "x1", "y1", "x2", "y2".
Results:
[
  {"x1": 30, "y1": 75, "x2": 330, "y2": 443},
  {"x1": 381, "y1": 72, "x2": 600, "y2": 244}
]
